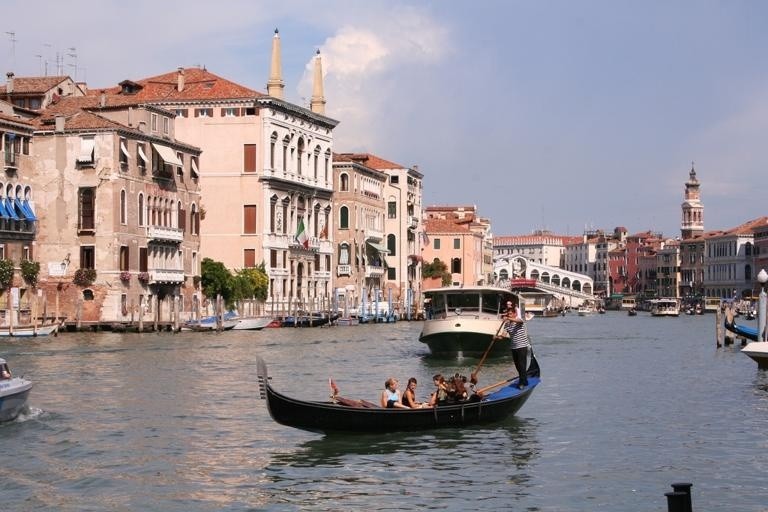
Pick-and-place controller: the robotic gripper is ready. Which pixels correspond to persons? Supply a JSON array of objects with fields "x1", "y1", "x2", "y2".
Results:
[
  {"x1": 381, "y1": 377, "x2": 410, "y2": 408},
  {"x1": 421, "y1": 374, "x2": 446, "y2": 408},
  {"x1": 493, "y1": 308, "x2": 530, "y2": 389},
  {"x1": 402, "y1": 376, "x2": 420, "y2": 407}
]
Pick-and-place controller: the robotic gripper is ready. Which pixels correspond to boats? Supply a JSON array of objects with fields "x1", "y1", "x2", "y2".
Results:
[
  {"x1": 578, "y1": 299, "x2": 605, "y2": 316},
  {"x1": 649, "y1": 298, "x2": 680, "y2": 316},
  {"x1": 256, "y1": 336, "x2": 541, "y2": 434},
  {"x1": 184, "y1": 310, "x2": 339, "y2": 330},
  {"x1": 0, "y1": 325, "x2": 59, "y2": 336},
  {"x1": 419, "y1": 286, "x2": 526, "y2": 355},
  {"x1": 0, "y1": 356, "x2": 34, "y2": 423}
]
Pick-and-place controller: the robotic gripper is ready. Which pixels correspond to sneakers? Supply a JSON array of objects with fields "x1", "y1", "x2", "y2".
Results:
[{"x1": 517, "y1": 383, "x2": 529, "y2": 390}]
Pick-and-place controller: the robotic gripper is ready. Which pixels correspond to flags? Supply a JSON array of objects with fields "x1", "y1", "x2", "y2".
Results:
[
  {"x1": 294, "y1": 218, "x2": 308, "y2": 250},
  {"x1": 423, "y1": 228, "x2": 430, "y2": 247}
]
